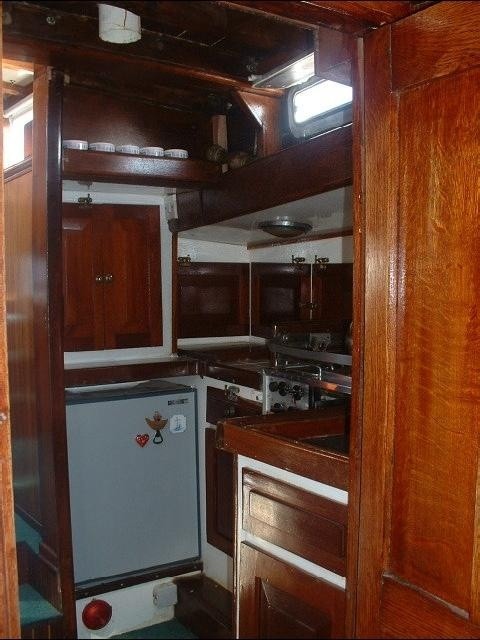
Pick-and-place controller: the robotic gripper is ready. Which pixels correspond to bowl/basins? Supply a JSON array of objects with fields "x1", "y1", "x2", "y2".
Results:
[{"x1": 62, "y1": 140, "x2": 188, "y2": 160}]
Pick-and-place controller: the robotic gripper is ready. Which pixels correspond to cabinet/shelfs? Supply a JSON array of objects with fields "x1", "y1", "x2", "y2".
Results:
[
  {"x1": 199, "y1": 386, "x2": 262, "y2": 558},
  {"x1": 250, "y1": 265, "x2": 351, "y2": 335},
  {"x1": 60, "y1": 201, "x2": 163, "y2": 351},
  {"x1": 234, "y1": 456, "x2": 357, "y2": 640}
]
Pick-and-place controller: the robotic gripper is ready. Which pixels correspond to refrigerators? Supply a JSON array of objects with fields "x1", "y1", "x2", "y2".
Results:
[{"x1": 64, "y1": 380, "x2": 202, "y2": 587}]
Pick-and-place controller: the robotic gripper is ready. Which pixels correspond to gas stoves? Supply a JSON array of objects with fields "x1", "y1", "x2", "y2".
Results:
[{"x1": 263, "y1": 360, "x2": 353, "y2": 416}]
